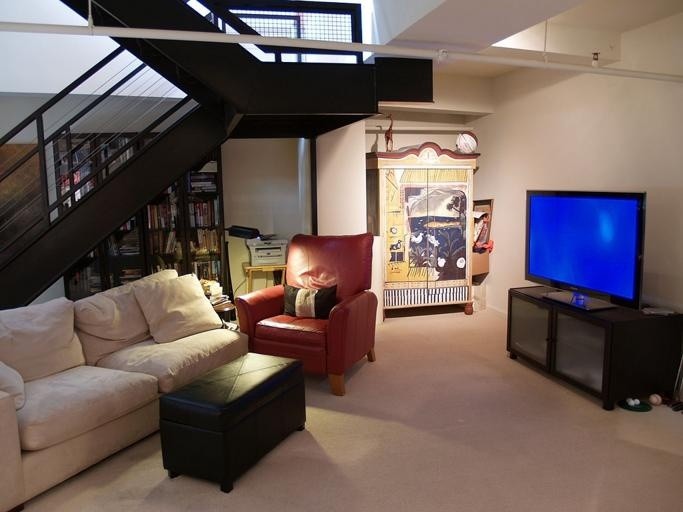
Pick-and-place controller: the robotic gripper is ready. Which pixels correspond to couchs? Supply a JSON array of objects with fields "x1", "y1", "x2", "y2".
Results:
[
  {"x1": 0, "y1": 269, "x2": 248, "y2": 511},
  {"x1": 233, "y1": 233, "x2": 377, "y2": 397}
]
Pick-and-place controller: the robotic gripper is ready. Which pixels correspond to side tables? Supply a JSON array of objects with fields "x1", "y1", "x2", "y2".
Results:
[{"x1": 210, "y1": 295, "x2": 234, "y2": 322}]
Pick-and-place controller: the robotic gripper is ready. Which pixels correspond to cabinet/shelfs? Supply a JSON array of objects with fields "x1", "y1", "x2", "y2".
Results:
[
  {"x1": 365, "y1": 142, "x2": 481, "y2": 322},
  {"x1": 51, "y1": 132, "x2": 227, "y2": 298},
  {"x1": 506, "y1": 285, "x2": 683, "y2": 411}
]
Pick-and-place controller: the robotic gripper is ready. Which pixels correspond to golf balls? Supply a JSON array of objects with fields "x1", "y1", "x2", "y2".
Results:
[
  {"x1": 628, "y1": 400, "x2": 634, "y2": 406},
  {"x1": 626, "y1": 398, "x2": 632, "y2": 403},
  {"x1": 634, "y1": 399, "x2": 640, "y2": 405}
]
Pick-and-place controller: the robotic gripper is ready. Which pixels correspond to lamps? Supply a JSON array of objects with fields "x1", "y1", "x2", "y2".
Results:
[
  {"x1": 591, "y1": 52, "x2": 599, "y2": 68},
  {"x1": 223, "y1": 224, "x2": 260, "y2": 239}
]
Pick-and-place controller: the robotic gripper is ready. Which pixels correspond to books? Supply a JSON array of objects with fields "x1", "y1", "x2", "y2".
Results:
[
  {"x1": 106, "y1": 216, "x2": 141, "y2": 257},
  {"x1": 109, "y1": 267, "x2": 143, "y2": 287},
  {"x1": 147, "y1": 178, "x2": 179, "y2": 230},
  {"x1": 150, "y1": 229, "x2": 179, "y2": 255},
  {"x1": 84, "y1": 246, "x2": 100, "y2": 259},
  {"x1": 190, "y1": 159, "x2": 218, "y2": 193},
  {"x1": 151, "y1": 261, "x2": 181, "y2": 275},
  {"x1": 69, "y1": 266, "x2": 102, "y2": 301},
  {"x1": 188, "y1": 195, "x2": 220, "y2": 228},
  {"x1": 188, "y1": 228, "x2": 221, "y2": 255},
  {"x1": 57, "y1": 137, "x2": 154, "y2": 209},
  {"x1": 191, "y1": 256, "x2": 223, "y2": 281},
  {"x1": 199, "y1": 278, "x2": 232, "y2": 307}
]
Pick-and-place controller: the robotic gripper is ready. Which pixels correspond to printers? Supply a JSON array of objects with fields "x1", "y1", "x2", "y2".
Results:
[{"x1": 246, "y1": 235, "x2": 288, "y2": 267}]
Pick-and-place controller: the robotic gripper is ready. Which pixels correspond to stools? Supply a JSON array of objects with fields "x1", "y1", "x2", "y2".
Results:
[{"x1": 159, "y1": 352, "x2": 306, "y2": 493}]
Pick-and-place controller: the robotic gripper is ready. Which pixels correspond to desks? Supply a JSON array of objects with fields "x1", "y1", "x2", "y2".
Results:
[{"x1": 232, "y1": 262, "x2": 286, "y2": 293}]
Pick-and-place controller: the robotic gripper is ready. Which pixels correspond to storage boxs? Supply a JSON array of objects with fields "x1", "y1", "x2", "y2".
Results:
[
  {"x1": 471, "y1": 250, "x2": 490, "y2": 275},
  {"x1": 472, "y1": 284, "x2": 487, "y2": 310}
]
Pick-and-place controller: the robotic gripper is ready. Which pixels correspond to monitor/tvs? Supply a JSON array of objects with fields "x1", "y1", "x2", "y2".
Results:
[{"x1": 526, "y1": 190, "x2": 648, "y2": 314}]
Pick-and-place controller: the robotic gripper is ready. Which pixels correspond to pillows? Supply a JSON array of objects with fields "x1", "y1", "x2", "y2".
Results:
[
  {"x1": 133, "y1": 273, "x2": 222, "y2": 345},
  {"x1": 283, "y1": 283, "x2": 336, "y2": 319}
]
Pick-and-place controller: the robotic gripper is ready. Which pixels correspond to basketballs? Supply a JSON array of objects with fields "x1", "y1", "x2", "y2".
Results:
[{"x1": 455, "y1": 131, "x2": 479, "y2": 154}]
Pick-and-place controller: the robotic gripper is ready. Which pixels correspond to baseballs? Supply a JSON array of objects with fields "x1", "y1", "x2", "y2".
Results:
[{"x1": 649, "y1": 394, "x2": 661, "y2": 406}]
